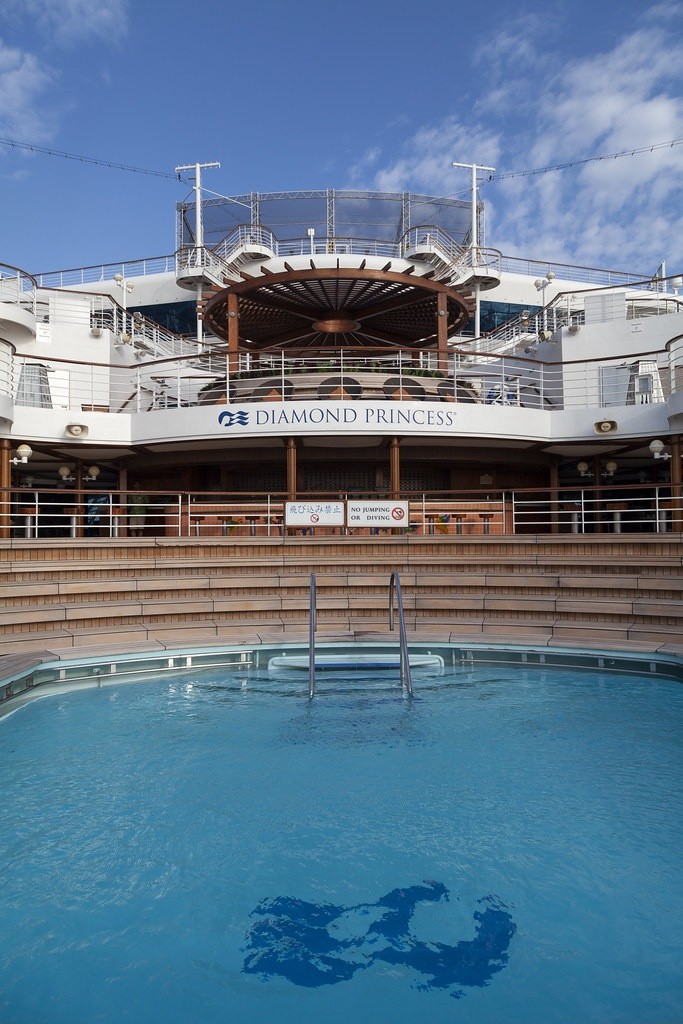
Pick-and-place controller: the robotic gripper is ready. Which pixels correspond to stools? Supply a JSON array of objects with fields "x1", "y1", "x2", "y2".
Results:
[
  {"x1": 452, "y1": 515, "x2": 466, "y2": 533},
  {"x1": 191, "y1": 516, "x2": 205, "y2": 536},
  {"x1": 245, "y1": 516, "x2": 261, "y2": 536},
  {"x1": 275, "y1": 516, "x2": 286, "y2": 537},
  {"x1": 425, "y1": 515, "x2": 438, "y2": 534},
  {"x1": 479, "y1": 514, "x2": 493, "y2": 533},
  {"x1": 218, "y1": 516, "x2": 232, "y2": 536}
]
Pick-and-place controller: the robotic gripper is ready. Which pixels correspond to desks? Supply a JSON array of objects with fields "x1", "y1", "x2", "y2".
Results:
[
  {"x1": 653, "y1": 502, "x2": 672, "y2": 532},
  {"x1": 606, "y1": 503, "x2": 628, "y2": 534},
  {"x1": 19, "y1": 507, "x2": 40, "y2": 538},
  {"x1": 104, "y1": 507, "x2": 126, "y2": 537},
  {"x1": 63, "y1": 508, "x2": 86, "y2": 537},
  {"x1": 564, "y1": 505, "x2": 582, "y2": 533}
]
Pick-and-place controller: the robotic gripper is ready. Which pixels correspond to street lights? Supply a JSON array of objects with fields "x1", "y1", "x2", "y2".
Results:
[
  {"x1": 534, "y1": 272, "x2": 556, "y2": 333},
  {"x1": 114, "y1": 273, "x2": 135, "y2": 334},
  {"x1": 650, "y1": 439, "x2": 683, "y2": 533},
  {"x1": 577, "y1": 460, "x2": 619, "y2": 534},
  {"x1": 58, "y1": 464, "x2": 100, "y2": 539},
  {"x1": 0, "y1": 444, "x2": 33, "y2": 538}
]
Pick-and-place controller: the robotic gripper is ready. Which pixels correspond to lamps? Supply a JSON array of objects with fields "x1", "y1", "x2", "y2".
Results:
[
  {"x1": 8, "y1": 444, "x2": 32, "y2": 465},
  {"x1": 540, "y1": 331, "x2": 556, "y2": 344},
  {"x1": 114, "y1": 334, "x2": 130, "y2": 349},
  {"x1": 577, "y1": 462, "x2": 595, "y2": 479},
  {"x1": 135, "y1": 349, "x2": 147, "y2": 358},
  {"x1": 82, "y1": 466, "x2": 100, "y2": 481},
  {"x1": 649, "y1": 440, "x2": 671, "y2": 461},
  {"x1": 58, "y1": 466, "x2": 76, "y2": 482},
  {"x1": 20, "y1": 476, "x2": 34, "y2": 489},
  {"x1": 601, "y1": 462, "x2": 616, "y2": 476}
]
